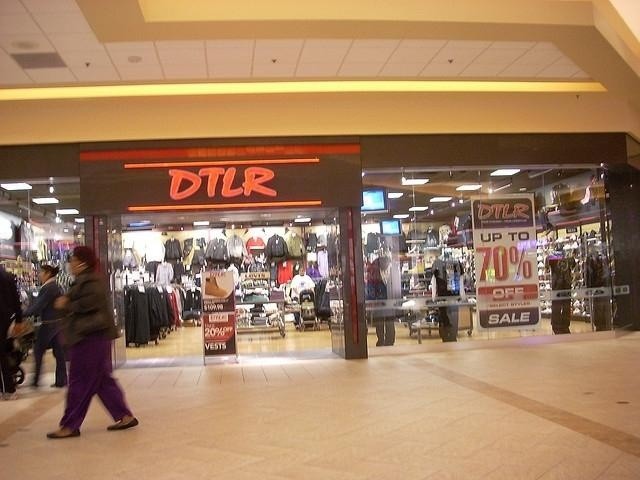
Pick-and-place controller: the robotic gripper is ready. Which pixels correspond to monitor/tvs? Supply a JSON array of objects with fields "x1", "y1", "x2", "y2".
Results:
[
  {"x1": 380, "y1": 218, "x2": 402, "y2": 237},
  {"x1": 360, "y1": 187, "x2": 390, "y2": 215}
]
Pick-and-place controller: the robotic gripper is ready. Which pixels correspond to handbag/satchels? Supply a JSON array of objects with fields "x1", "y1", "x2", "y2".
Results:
[{"x1": 8, "y1": 321, "x2": 33, "y2": 339}]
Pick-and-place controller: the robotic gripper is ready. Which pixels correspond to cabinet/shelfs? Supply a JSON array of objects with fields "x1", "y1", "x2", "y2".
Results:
[
  {"x1": 407, "y1": 301, "x2": 473, "y2": 336},
  {"x1": 237, "y1": 301, "x2": 287, "y2": 337}
]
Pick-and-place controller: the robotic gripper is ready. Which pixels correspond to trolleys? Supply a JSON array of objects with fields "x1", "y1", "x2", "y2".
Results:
[{"x1": 8, "y1": 330, "x2": 35, "y2": 384}]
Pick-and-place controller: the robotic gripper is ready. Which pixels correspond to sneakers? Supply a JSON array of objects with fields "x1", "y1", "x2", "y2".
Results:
[
  {"x1": 106, "y1": 416, "x2": 139, "y2": 431},
  {"x1": 47, "y1": 429, "x2": 81, "y2": 440}
]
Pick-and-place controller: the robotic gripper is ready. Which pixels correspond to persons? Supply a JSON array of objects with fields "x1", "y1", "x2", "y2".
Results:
[
  {"x1": 25, "y1": 264, "x2": 69, "y2": 390},
  {"x1": 289, "y1": 264, "x2": 316, "y2": 329},
  {"x1": 545, "y1": 249, "x2": 580, "y2": 334},
  {"x1": 47, "y1": 245, "x2": 140, "y2": 439},
  {"x1": 0, "y1": 268, "x2": 26, "y2": 401},
  {"x1": 430, "y1": 248, "x2": 467, "y2": 342},
  {"x1": 584, "y1": 240, "x2": 613, "y2": 333},
  {"x1": 366, "y1": 254, "x2": 401, "y2": 346}
]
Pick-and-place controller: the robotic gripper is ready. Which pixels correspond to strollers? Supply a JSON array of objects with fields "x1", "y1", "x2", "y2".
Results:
[{"x1": 297, "y1": 289, "x2": 321, "y2": 332}]
